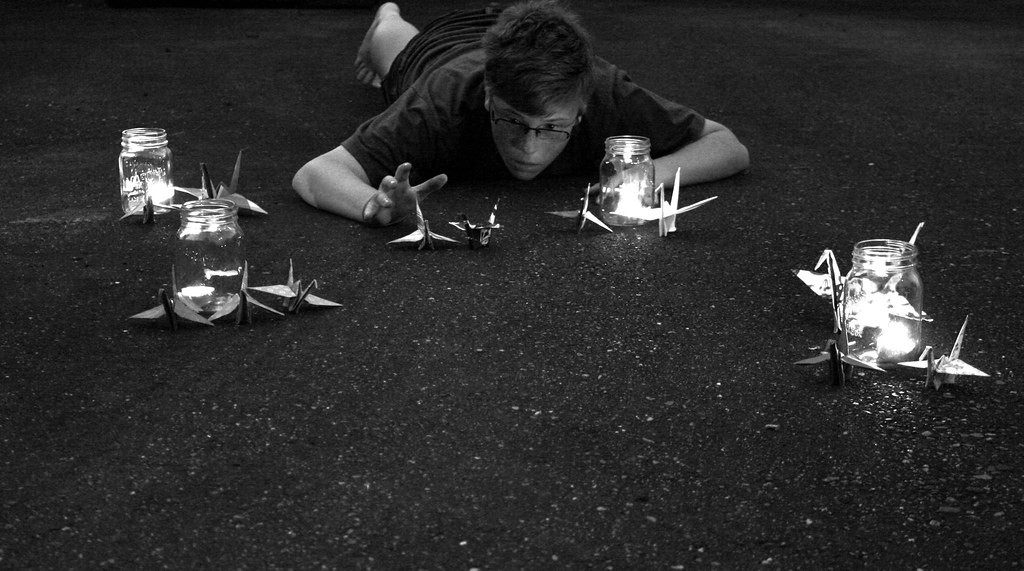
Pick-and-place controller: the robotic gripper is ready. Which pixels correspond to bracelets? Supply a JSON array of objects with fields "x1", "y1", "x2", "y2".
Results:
[{"x1": 361, "y1": 190, "x2": 382, "y2": 219}]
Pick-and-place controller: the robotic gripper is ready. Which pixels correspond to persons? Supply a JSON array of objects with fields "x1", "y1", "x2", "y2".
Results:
[{"x1": 292, "y1": 0, "x2": 749, "y2": 227}]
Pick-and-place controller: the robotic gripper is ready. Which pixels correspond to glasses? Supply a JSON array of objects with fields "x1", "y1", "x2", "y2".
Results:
[{"x1": 490, "y1": 98, "x2": 583, "y2": 142}]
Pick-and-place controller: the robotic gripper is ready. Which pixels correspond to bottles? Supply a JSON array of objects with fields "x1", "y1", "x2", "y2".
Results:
[
  {"x1": 118, "y1": 128, "x2": 176, "y2": 216},
  {"x1": 174, "y1": 198, "x2": 248, "y2": 316},
  {"x1": 845, "y1": 239, "x2": 923, "y2": 370},
  {"x1": 598, "y1": 135, "x2": 656, "y2": 227}
]
